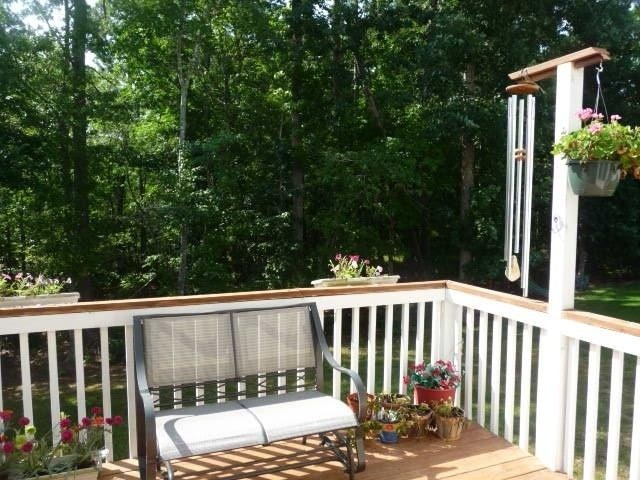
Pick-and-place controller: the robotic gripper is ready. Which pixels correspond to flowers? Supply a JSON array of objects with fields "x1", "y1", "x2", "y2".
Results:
[
  {"x1": 403, "y1": 360, "x2": 461, "y2": 388},
  {"x1": 0, "y1": 272, "x2": 72, "y2": 296},
  {"x1": 550, "y1": 109, "x2": 640, "y2": 178},
  {"x1": 0, "y1": 406, "x2": 122, "y2": 473},
  {"x1": 326, "y1": 253, "x2": 383, "y2": 277}
]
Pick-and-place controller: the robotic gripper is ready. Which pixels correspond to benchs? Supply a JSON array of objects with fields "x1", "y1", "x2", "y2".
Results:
[{"x1": 133, "y1": 301, "x2": 368, "y2": 480}]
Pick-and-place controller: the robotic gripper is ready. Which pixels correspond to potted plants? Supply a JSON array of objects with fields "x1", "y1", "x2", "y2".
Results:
[{"x1": 348, "y1": 391, "x2": 463, "y2": 443}]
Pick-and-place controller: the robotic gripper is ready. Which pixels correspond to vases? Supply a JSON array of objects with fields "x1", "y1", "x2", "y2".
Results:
[
  {"x1": 309, "y1": 275, "x2": 401, "y2": 288},
  {"x1": 565, "y1": 161, "x2": 621, "y2": 196},
  {"x1": 2, "y1": 291, "x2": 80, "y2": 305},
  {"x1": 416, "y1": 388, "x2": 455, "y2": 406},
  {"x1": 24, "y1": 468, "x2": 98, "y2": 479}
]
